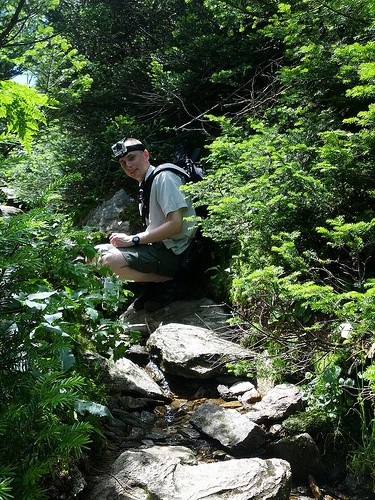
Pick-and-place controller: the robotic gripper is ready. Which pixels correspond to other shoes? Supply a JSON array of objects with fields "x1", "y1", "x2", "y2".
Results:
[{"x1": 156, "y1": 277, "x2": 183, "y2": 303}]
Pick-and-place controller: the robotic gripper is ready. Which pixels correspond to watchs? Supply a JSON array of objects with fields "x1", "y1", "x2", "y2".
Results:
[{"x1": 132, "y1": 235, "x2": 140, "y2": 247}]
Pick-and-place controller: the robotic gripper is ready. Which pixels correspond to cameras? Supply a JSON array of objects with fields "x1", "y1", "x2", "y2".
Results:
[{"x1": 111, "y1": 140, "x2": 127, "y2": 157}]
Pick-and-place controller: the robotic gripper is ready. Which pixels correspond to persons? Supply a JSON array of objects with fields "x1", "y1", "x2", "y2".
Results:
[{"x1": 87, "y1": 138, "x2": 200, "y2": 313}]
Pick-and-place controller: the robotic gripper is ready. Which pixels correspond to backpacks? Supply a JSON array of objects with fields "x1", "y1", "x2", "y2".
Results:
[{"x1": 142, "y1": 158, "x2": 213, "y2": 255}]
